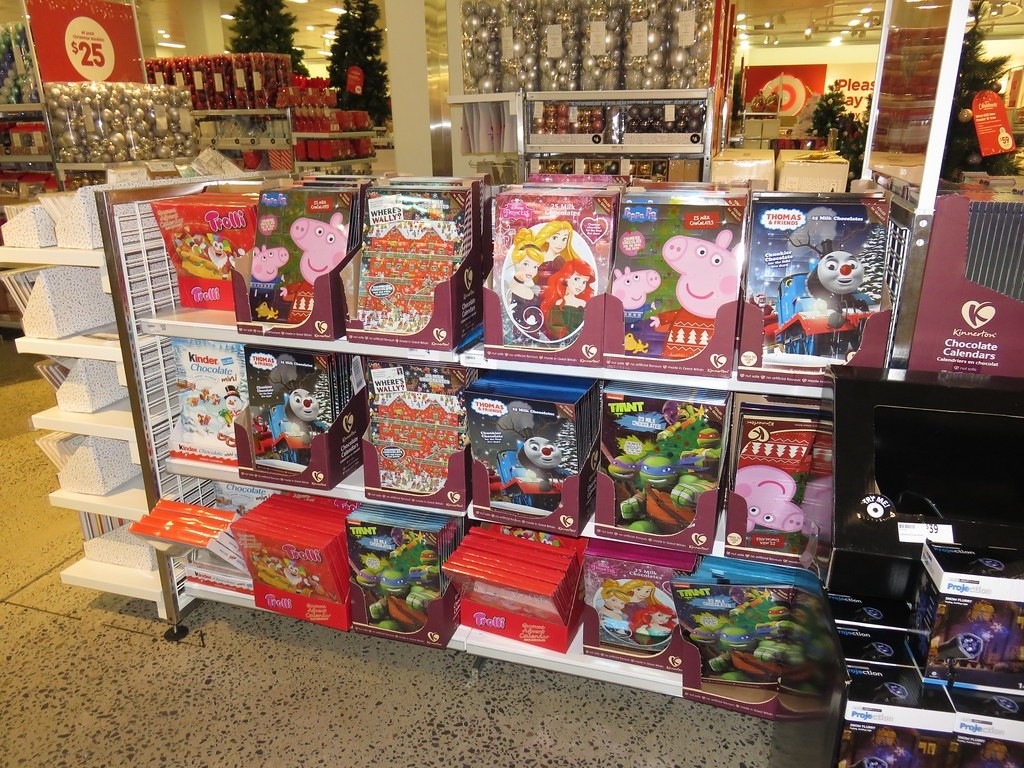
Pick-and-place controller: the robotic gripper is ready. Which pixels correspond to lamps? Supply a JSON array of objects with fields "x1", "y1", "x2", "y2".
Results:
[
  {"x1": 764, "y1": 17, "x2": 774, "y2": 28},
  {"x1": 858, "y1": 31, "x2": 866, "y2": 38},
  {"x1": 772, "y1": 36, "x2": 779, "y2": 45},
  {"x1": 872, "y1": 18, "x2": 880, "y2": 26},
  {"x1": 804, "y1": 23, "x2": 814, "y2": 35},
  {"x1": 764, "y1": 35, "x2": 769, "y2": 44},
  {"x1": 851, "y1": 29, "x2": 859, "y2": 36},
  {"x1": 864, "y1": 16, "x2": 875, "y2": 29}
]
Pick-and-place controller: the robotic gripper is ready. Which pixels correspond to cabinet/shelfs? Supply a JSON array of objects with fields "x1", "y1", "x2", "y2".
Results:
[
  {"x1": 446, "y1": 0, "x2": 737, "y2": 183},
  {"x1": 0, "y1": 0, "x2": 198, "y2": 329},
  {"x1": 860, "y1": 0, "x2": 1024, "y2": 388},
  {"x1": 517, "y1": 85, "x2": 724, "y2": 185},
  {"x1": 192, "y1": 106, "x2": 378, "y2": 179},
  {"x1": 0, "y1": 147, "x2": 1024, "y2": 768}
]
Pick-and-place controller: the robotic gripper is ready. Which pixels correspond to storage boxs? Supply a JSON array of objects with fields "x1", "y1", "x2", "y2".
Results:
[{"x1": 128, "y1": 116, "x2": 886, "y2": 692}]
